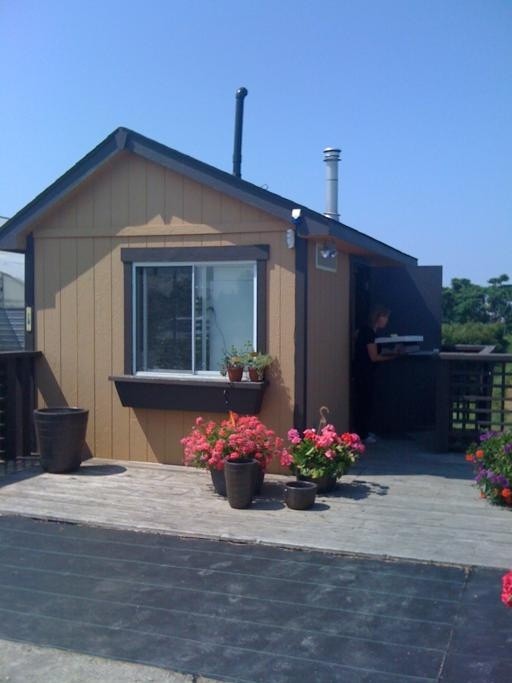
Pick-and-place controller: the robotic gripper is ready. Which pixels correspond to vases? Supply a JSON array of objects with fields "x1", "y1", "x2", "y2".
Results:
[
  {"x1": 296, "y1": 467, "x2": 336, "y2": 493},
  {"x1": 224, "y1": 460, "x2": 257, "y2": 508},
  {"x1": 208, "y1": 463, "x2": 265, "y2": 496},
  {"x1": 34, "y1": 408, "x2": 87, "y2": 473},
  {"x1": 285, "y1": 481, "x2": 317, "y2": 509},
  {"x1": 227, "y1": 366, "x2": 243, "y2": 382},
  {"x1": 248, "y1": 367, "x2": 263, "y2": 382}
]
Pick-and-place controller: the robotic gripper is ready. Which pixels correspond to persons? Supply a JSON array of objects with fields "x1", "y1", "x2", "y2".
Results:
[{"x1": 355, "y1": 306, "x2": 404, "y2": 444}]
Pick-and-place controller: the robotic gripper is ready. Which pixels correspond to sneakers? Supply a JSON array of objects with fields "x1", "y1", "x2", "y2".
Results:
[{"x1": 361, "y1": 431, "x2": 377, "y2": 445}]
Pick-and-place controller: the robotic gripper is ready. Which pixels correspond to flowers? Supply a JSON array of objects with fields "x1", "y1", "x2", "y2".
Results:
[
  {"x1": 464, "y1": 429, "x2": 512, "y2": 507},
  {"x1": 500, "y1": 570, "x2": 512, "y2": 607},
  {"x1": 245, "y1": 341, "x2": 273, "y2": 370},
  {"x1": 179, "y1": 411, "x2": 283, "y2": 472},
  {"x1": 277, "y1": 425, "x2": 365, "y2": 479},
  {"x1": 217, "y1": 344, "x2": 247, "y2": 377}
]
